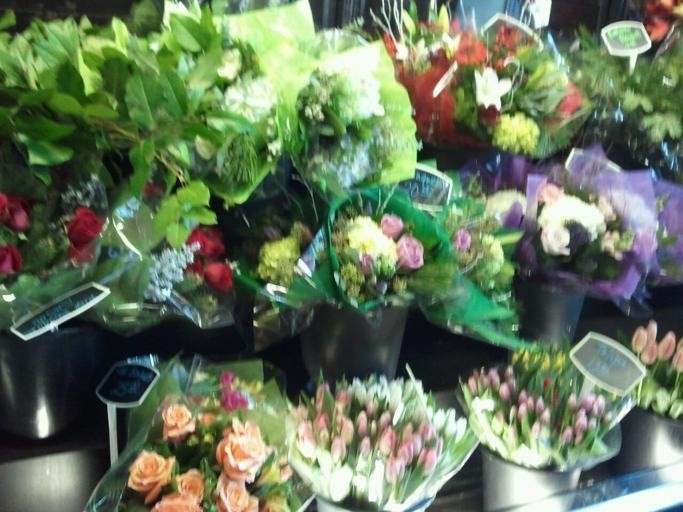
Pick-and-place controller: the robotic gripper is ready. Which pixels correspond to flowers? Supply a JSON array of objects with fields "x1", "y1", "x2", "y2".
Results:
[{"x1": 1, "y1": 1, "x2": 683, "y2": 511}]
